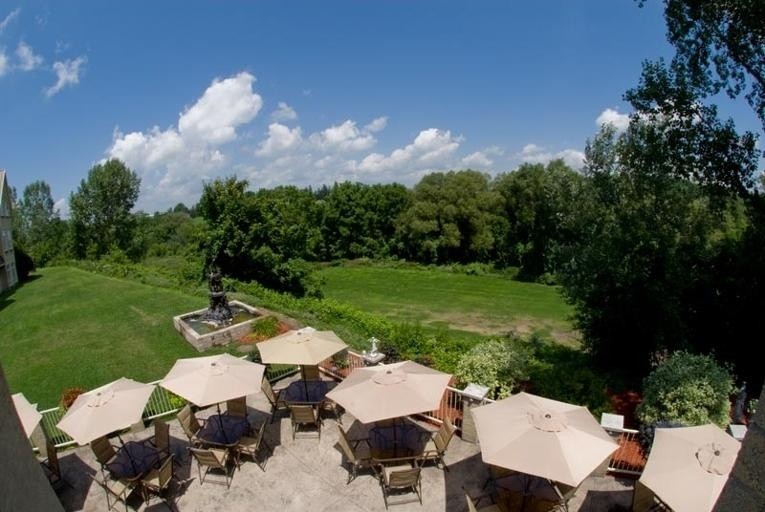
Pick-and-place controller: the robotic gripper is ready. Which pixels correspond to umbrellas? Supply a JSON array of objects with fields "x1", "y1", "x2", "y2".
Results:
[
  {"x1": 255, "y1": 326, "x2": 350, "y2": 396},
  {"x1": 54, "y1": 374, "x2": 157, "y2": 460},
  {"x1": 157, "y1": 352, "x2": 266, "y2": 427},
  {"x1": 11, "y1": 392, "x2": 43, "y2": 442},
  {"x1": 323, "y1": 359, "x2": 455, "y2": 438},
  {"x1": 638, "y1": 421, "x2": 765, "y2": 512},
  {"x1": 470, "y1": 390, "x2": 622, "y2": 487}
]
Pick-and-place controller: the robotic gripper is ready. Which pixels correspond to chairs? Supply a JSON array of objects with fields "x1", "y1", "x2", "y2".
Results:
[
  {"x1": 86, "y1": 420, "x2": 196, "y2": 512},
  {"x1": 177, "y1": 396, "x2": 270, "y2": 489},
  {"x1": 462, "y1": 465, "x2": 569, "y2": 512},
  {"x1": 261, "y1": 364, "x2": 340, "y2": 444},
  {"x1": 339, "y1": 417, "x2": 457, "y2": 510}
]
